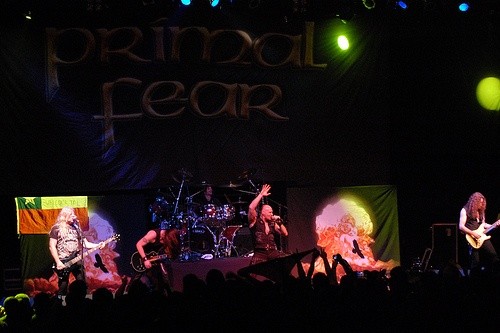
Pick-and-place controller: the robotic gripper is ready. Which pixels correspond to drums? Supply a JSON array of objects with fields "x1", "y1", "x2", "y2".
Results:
[
  {"x1": 219, "y1": 225, "x2": 255, "y2": 257},
  {"x1": 182, "y1": 224, "x2": 217, "y2": 258},
  {"x1": 201, "y1": 204, "x2": 219, "y2": 218},
  {"x1": 149, "y1": 197, "x2": 175, "y2": 220},
  {"x1": 220, "y1": 204, "x2": 235, "y2": 221}
]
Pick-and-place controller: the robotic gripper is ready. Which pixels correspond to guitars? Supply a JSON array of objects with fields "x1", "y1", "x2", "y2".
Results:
[
  {"x1": 466, "y1": 220, "x2": 500, "y2": 249},
  {"x1": 53, "y1": 234, "x2": 120, "y2": 280},
  {"x1": 132, "y1": 247, "x2": 190, "y2": 272}
]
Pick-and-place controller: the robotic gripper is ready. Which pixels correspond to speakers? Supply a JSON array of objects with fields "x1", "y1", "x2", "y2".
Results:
[{"x1": 419, "y1": 224, "x2": 458, "y2": 271}]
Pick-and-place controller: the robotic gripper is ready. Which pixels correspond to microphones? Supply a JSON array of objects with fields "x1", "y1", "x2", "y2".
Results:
[
  {"x1": 249, "y1": 180, "x2": 258, "y2": 191},
  {"x1": 276, "y1": 219, "x2": 283, "y2": 223},
  {"x1": 73, "y1": 219, "x2": 80, "y2": 223}
]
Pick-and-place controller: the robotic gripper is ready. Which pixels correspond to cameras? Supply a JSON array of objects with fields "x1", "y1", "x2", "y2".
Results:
[{"x1": 333, "y1": 255, "x2": 339, "y2": 260}]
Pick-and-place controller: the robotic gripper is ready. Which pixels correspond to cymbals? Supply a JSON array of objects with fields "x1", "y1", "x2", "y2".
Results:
[
  {"x1": 220, "y1": 183, "x2": 242, "y2": 187},
  {"x1": 233, "y1": 201, "x2": 250, "y2": 203},
  {"x1": 172, "y1": 171, "x2": 193, "y2": 183},
  {"x1": 185, "y1": 203, "x2": 200, "y2": 206}
]
